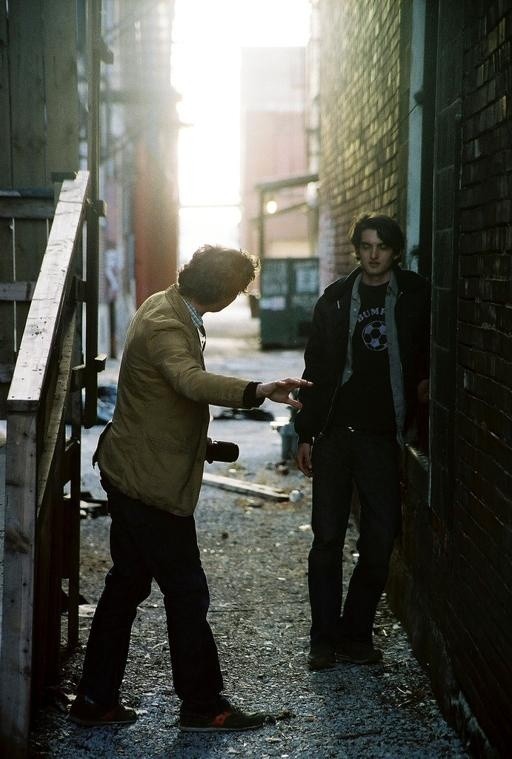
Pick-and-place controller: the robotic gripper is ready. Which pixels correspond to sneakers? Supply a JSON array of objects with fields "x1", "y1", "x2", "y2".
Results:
[
  {"x1": 307, "y1": 646, "x2": 383, "y2": 672},
  {"x1": 179, "y1": 694, "x2": 265, "y2": 731},
  {"x1": 69, "y1": 697, "x2": 137, "y2": 725}
]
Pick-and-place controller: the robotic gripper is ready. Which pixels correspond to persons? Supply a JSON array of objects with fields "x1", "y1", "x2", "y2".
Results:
[
  {"x1": 67, "y1": 241, "x2": 318, "y2": 735},
  {"x1": 287, "y1": 210, "x2": 434, "y2": 670}
]
